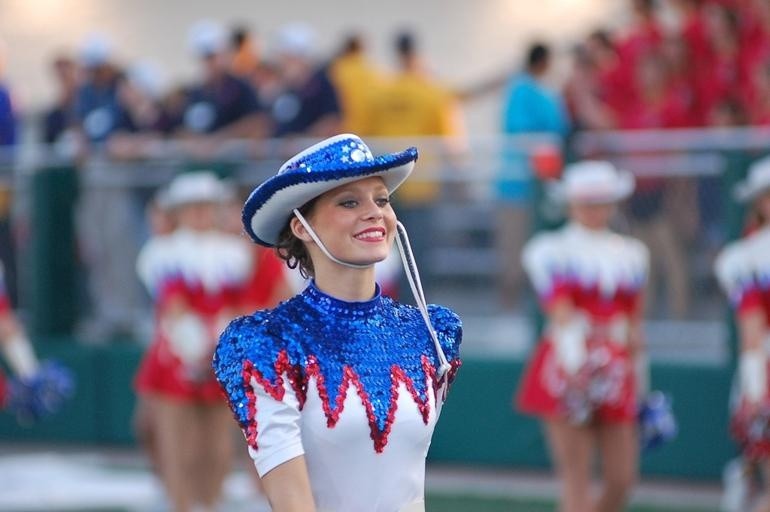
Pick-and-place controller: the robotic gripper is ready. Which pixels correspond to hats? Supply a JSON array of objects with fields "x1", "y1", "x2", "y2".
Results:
[
  {"x1": 548, "y1": 159, "x2": 638, "y2": 205},
  {"x1": 241, "y1": 133, "x2": 419, "y2": 248},
  {"x1": 158, "y1": 170, "x2": 233, "y2": 208},
  {"x1": 730, "y1": 155, "x2": 770, "y2": 207}
]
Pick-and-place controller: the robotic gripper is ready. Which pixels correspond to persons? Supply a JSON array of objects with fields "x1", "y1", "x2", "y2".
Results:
[
  {"x1": 519, "y1": 161, "x2": 651, "y2": 512},
  {"x1": 710, "y1": 160, "x2": 770, "y2": 512},
  {"x1": 214, "y1": 131, "x2": 461, "y2": 512},
  {"x1": 135, "y1": 172, "x2": 286, "y2": 512},
  {"x1": 0, "y1": 301, "x2": 72, "y2": 427}
]
